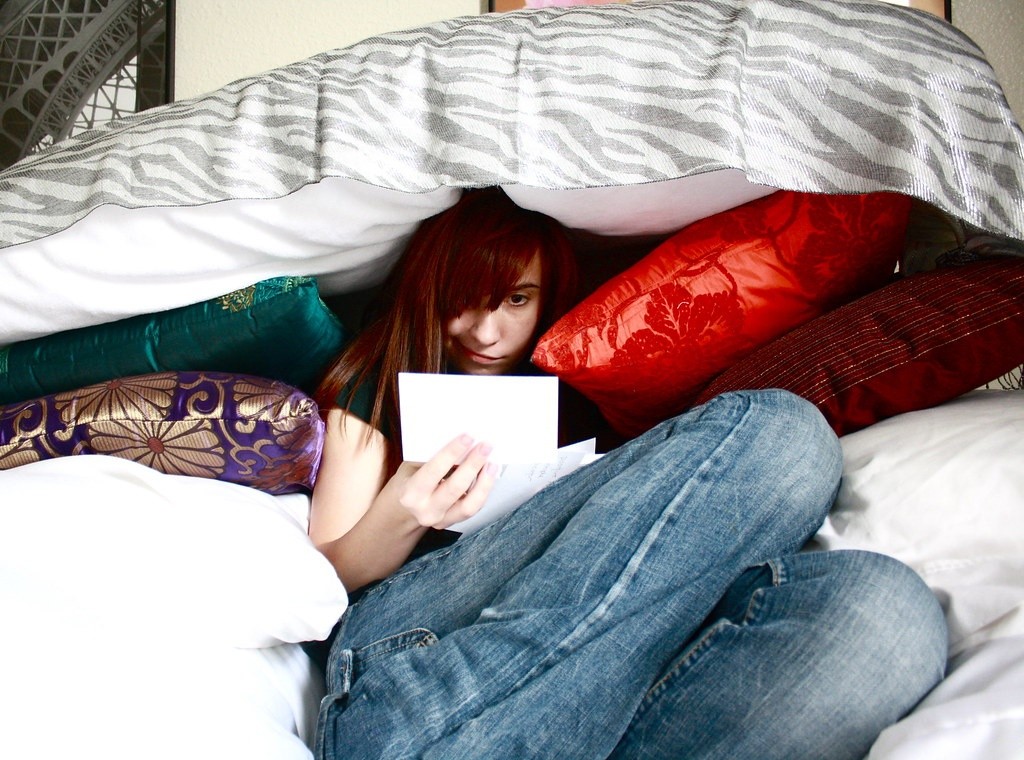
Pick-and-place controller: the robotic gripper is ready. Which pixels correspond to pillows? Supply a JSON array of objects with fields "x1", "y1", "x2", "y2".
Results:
[
  {"x1": 0, "y1": 0, "x2": 1024, "y2": 344},
  {"x1": 0, "y1": 454, "x2": 350, "y2": 760},
  {"x1": 0, "y1": 275, "x2": 357, "y2": 493},
  {"x1": 529, "y1": 192, "x2": 1024, "y2": 437},
  {"x1": 814, "y1": 390, "x2": 1022, "y2": 760}
]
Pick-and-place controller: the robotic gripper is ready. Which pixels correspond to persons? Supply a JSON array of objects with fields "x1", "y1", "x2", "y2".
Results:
[{"x1": 307, "y1": 188, "x2": 948, "y2": 760}]
